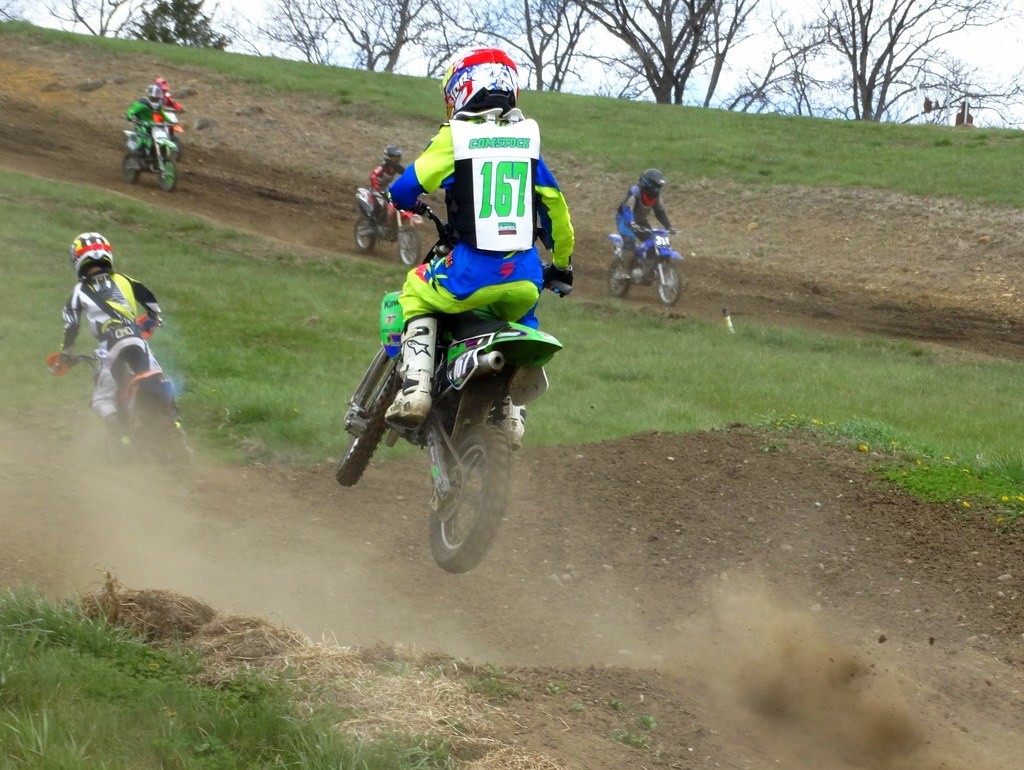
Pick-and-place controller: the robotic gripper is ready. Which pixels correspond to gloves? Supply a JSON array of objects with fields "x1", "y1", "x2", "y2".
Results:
[
  {"x1": 636, "y1": 226, "x2": 650, "y2": 242},
  {"x1": 55, "y1": 353, "x2": 76, "y2": 369},
  {"x1": 665, "y1": 224, "x2": 675, "y2": 234},
  {"x1": 397, "y1": 199, "x2": 421, "y2": 214},
  {"x1": 542, "y1": 264, "x2": 573, "y2": 298},
  {"x1": 174, "y1": 103, "x2": 184, "y2": 112},
  {"x1": 380, "y1": 189, "x2": 392, "y2": 202},
  {"x1": 145, "y1": 316, "x2": 160, "y2": 330}
]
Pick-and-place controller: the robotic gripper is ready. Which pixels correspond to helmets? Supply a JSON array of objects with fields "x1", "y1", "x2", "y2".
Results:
[
  {"x1": 440, "y1": 49, "x2": 519, "y2": 121},
  {"x1": 383, "y1": 145, "x2": 401, "y2": 165},
  {"x1": 155, "y1": 78, "x2": 170, "y2": 91},
  {"x1": 71, "y1": 232, "x2": 113, "y2": 279},
  {"x1": 143, "y1": 85, "x2": 163, "y2": 110},
  {"x1": 640, "y1": 169, "x2": 666, "y2": 196}
]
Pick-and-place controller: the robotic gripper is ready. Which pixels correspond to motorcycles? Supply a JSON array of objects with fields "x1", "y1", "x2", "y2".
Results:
[
  {"x1": 354, "y1": 185, "x2": 426, "y2": 269},
  {"x1": 334, "y1": 188, "x2": 574, "y2": 574},
  {"x1": 49, "y1": 310, "x2": 195, "y2": 492},
  {"x1": 119, "y1": 106, "x2": 186, "y2": 193},
  {"x1": 606, "y1": 223, "x2": 685, "y2": 307}
]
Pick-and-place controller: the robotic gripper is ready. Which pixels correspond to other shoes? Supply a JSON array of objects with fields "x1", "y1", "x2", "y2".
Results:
[{"x1": 371, "y1": 212, "x2": 379, "y2": 223}]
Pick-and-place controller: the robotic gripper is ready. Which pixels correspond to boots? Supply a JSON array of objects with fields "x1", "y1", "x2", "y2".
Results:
[
  {"x1": 103, "y1": 411, "x2": 132, "y2": 451},
  {"x1": 621, "y1": 249, "x2": 633, "y2": 280},
  {"x1": 384, "y1": 313, "x2": 437, "y2": 430},
  {"x1": 486, "y1": 395, "x2": 526, "y2": 450}
]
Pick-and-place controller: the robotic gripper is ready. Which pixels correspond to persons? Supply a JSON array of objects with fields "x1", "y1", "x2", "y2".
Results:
[
  {"x1": 124, "y1": 78, "x2": 183, "y2": 158},
  {"x1": 614, "y1": 168, "x2": 678, "y2": 282},
  {"x1": 384, "y1": 45, "x2": 575, "y2": 452},
  {"x1": 54, "y1": 231, "x2": 188, "y2": 450},
  {"x1": 370, "y1": 145, "x2": 405, "y2": 233}
]
